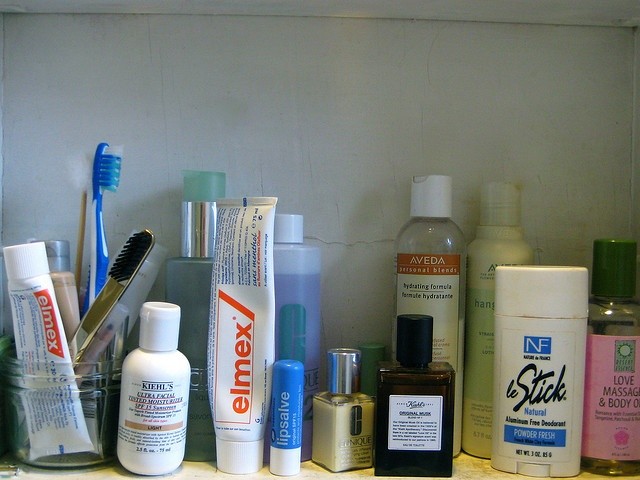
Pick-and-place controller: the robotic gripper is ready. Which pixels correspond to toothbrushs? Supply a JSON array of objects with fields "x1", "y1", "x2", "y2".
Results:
[
  {"x1": 70, "y1": 228, "x2": 156, "y2": 361},
  {"x1": 81, "y1": 142, "x2": 122, "y2": 319},
  {"x1": 71, "y1": 149, "x2": 93, "y2": 301}
]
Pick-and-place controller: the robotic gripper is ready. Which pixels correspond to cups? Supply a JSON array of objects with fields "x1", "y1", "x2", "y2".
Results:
[{"x1": 1, "y1": 346, "x2": 116, "y2": 466}]
